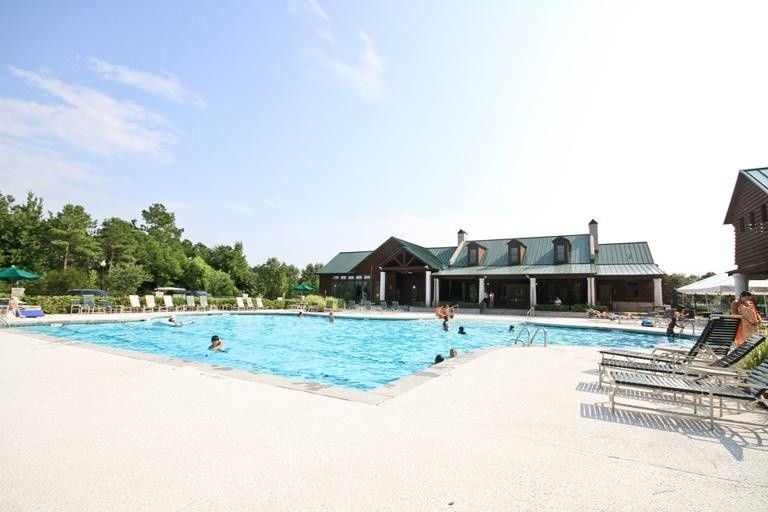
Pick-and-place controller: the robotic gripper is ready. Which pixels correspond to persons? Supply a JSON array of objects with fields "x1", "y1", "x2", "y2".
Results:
[
  {"x1": 478, "y1": 290, "x2": 487, "y2": 314},
  {"x1": 552, "y1": 295, "x2": 562, "y2": 304},
  {"x1": 209, "y1": 334, "x2": 225, "y2": 352},
  {"x1": 297, "y1": 311, "x2": 304, "y2": 318},
  {"x1": 436, "y1": 303, "x2": 455, "y2": 319},
  {"x1": 650, "y1": 306, "x2": 661, "y2": 326},
  {"x1": 666, "y1": 317, "x2": 680, "y2": 337},
  {"x1": 683, "y1": 307, "x2": 689, "y2": 318},
  {"x1": 428, "y1": 354, "x2": 444, "y2": 368},
  {"x1": 443, "y1": 315, "x2": 450, "y2": 331},
  {"x1": 508, "y1": 325, "x2": 515, "y2": 332},
  {"x1": 448, "y1": 347, "x2": 458, "y2": 358},
  {"x1": 168, "y1": 316, "x2": 196, "y2": 326},
  {"x1": 328, "y1": 311, "x2": 335, "y2": 322},
  {"x1": 730, "y1": 291, "x2": 761, "y2": 348},
  {"x1": 589, "y1": 308, "x2": 608, "y2": 318},
  {"x1": 488, "y1": 290, "x2": 496, "y2": 310},
  {"x1": 458, "y1": 326, "x2": 467, "y2": 335}
]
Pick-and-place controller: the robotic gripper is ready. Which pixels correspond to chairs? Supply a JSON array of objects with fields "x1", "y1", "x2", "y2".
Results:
[
  {"x1": 598, "y1": 317, "x2": 768, "y2": 433},
  {"x1": 0, "y1": 287, "x2": 44, "y2": 319}
]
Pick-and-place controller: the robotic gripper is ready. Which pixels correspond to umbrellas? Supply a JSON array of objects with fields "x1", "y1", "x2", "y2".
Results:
[
  {"x1": 0, "y1": 267, "x2": 40, "y2": 287},
  {"x1": 288, "y1": 283, "x2": 317, "y2": 296}
]
